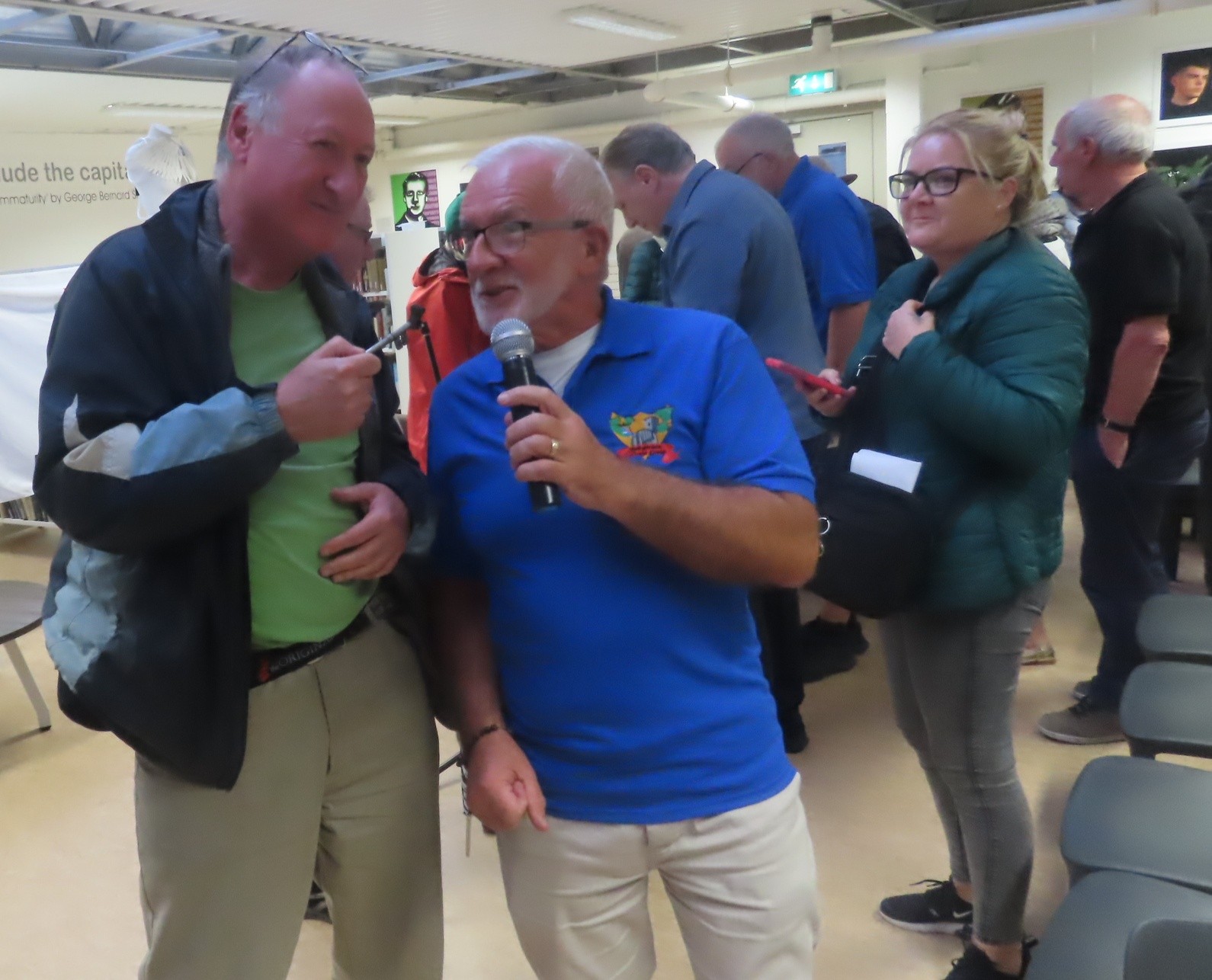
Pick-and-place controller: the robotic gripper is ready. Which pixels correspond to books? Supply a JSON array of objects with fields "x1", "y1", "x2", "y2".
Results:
[{"x1": 348, "y1": 251, "x2": 397, "y2": 349}]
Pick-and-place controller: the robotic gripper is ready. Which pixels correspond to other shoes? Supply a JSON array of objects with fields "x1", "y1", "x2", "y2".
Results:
[
  {"x1": 303, "y1": 880, "x2": 334, "y2": 926},
  {"x1": 777, "y1": 709, "x2": 809, "y2": 754},
  {"x1": 799, "y1": 612, "x2": 870, "y2": 684},
  {"x1": 1021, "y1": 645, "x2": 1056, "y2": 665}
]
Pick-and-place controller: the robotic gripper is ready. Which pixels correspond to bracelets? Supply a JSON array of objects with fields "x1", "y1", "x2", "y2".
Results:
[
  {"x1": 1096, "y1": 410, "x2": 1134, "y2": 434},
  {"x1": 457, "y1": 723, "x2": 510, "y2": 769}
]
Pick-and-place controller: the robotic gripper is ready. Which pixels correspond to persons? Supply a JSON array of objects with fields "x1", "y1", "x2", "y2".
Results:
[
  {"x1": 1161, "y1": 55, "x2": 1211, "y2": 120},
  {"x1": 370, "y1": 92, "x2": 1212, "y2": 752},
  {"x1": 32, "y1": 36, "x2": 452, "y2": 980},
  {"x1": 425, "y1": 135, "x2": 821, "y2": 980}
]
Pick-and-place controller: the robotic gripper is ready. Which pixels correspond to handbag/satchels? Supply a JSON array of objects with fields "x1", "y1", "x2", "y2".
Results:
[{"x1": 794, "y1": 455, "x2": 939, "y2": 620}]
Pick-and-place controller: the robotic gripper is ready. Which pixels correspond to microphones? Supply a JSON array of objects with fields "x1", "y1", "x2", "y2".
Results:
[{"x1": 489, "y1": 318, "x2": 562, "y2": 506}]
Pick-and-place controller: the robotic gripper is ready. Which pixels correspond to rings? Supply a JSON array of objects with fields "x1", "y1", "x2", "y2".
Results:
[{"x1": 548, "y1": 437, "x2": 559, "y2": 459}]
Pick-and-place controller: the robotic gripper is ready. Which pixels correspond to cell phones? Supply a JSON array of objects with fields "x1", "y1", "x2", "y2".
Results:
[{"x1": 764, "y1": 358, "x2": 848, "y2": 397}]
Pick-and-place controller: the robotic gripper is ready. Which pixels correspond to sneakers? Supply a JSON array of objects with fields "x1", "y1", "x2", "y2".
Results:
[
  {"x1": 1072, "y1": 676, "x2": 1100, "y2": 701},
  {"x1": 1037, "y1": 695, "x2": 1128, "y2": 744},
  {"x1": 944, "y1": 931, "x2": 1040, "y2": 980},
  {"x1": 880, "y1": 879, "x2": 974, "y2": 933}
]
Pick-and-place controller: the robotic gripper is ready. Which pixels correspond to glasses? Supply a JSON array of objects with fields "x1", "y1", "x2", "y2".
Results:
[
  {"x1": 243, "y1": 31, "x2": 371, "y2": 86},
  {"x1": 447, "y1": 218, "x2": 590, "y2": 261},
  {"x1": 889, "y1": 166, "x2": 1005, "y2": 200}
]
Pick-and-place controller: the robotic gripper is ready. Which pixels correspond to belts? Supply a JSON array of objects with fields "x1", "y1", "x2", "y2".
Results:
[{"x1": 249, "y1": 610, "x2": 371, "y2": 691}]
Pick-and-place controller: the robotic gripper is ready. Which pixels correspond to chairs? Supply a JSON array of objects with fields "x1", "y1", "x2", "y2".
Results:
[
  {"x1": 1016, "y1": 589, "x2": 1212, "y2": 980},
  {"x1": 0, "y1": 513, "x2": 59, "y2": 739}
]
